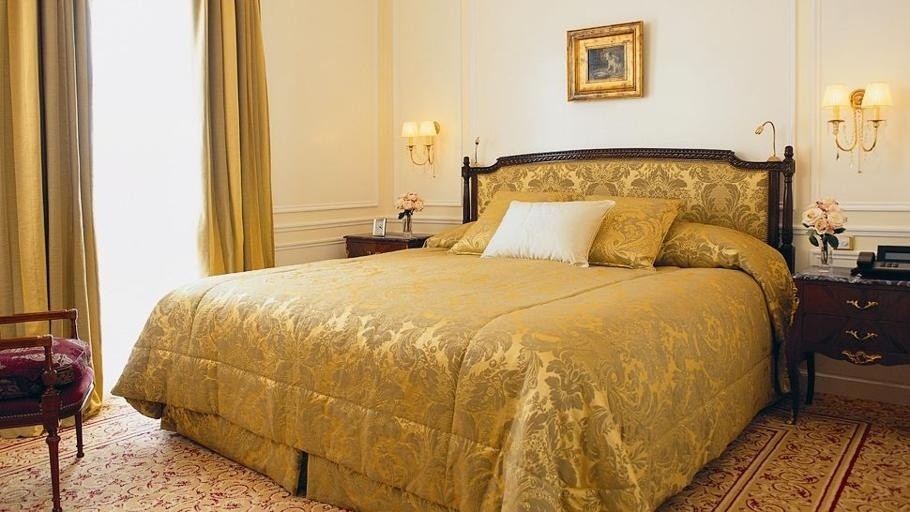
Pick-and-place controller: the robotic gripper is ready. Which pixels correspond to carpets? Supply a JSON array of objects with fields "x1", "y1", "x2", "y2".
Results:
[{"x1": 0, "y1": 381, "x2": 910, "y2": 512}]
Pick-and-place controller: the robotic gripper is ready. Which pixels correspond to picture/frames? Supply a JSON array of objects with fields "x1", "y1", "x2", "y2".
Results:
[
  {"x1": 372, "y1": 218, "x2": 386, "y2": 239},
  {"x1": 565, "y1": 20, "x2": 645, "y2": 100}
]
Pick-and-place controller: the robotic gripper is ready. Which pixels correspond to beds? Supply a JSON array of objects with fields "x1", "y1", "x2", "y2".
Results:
[{"x1": 113, "y1": 145, "x2": 796, "y2": 509}]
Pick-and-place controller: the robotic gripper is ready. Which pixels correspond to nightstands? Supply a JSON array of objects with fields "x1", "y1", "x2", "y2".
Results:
[
  {"x1": 342, "y1": 231, "x2": 433, "y2": 261},
  {"x1": 788, "y1": 264, "x2": 910, "y2": 427}
]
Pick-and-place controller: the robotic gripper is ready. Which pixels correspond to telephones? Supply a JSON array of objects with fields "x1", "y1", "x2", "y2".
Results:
[{"x1": 851, "y1": 246, "x2": 910, "y2": 280}]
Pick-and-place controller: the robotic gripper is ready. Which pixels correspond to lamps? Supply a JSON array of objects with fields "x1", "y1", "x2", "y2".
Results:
[
  {"x1": 820, "y1": 82, "x2": 896, "y2": 174},
  {"x1": 753, "y1": 120, "x2": 781, "y2": 160},
  {"x1": 400, "y1": 119, "x2": 440, "y2": 180}
]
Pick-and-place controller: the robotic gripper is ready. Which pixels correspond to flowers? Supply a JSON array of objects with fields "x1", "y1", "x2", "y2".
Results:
[
  {"x1": 396, "y1": 192, "x2": 423, "y2": 217},
  {"x1": 801, "y1": 198, "x2": 850, "y2": 264}
]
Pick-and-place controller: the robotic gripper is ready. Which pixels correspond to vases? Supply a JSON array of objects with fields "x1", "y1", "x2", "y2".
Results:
[
  {"x1": 400, "y1": 215, "x2": 412, "y2": 238},
  {"x1": 810, "y1": 246, "x2": 836, "y2": 279}
]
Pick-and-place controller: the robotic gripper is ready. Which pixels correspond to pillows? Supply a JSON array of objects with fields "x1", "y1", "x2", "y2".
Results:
[
  {"x1": 446, "y1": 189, "x2": 576, "y2": 259},
  {"x1": 479, "y1": 198, "x2": 618, "y2": 267},
  {"x1": 568, "y1": 195, "x2": 681, "y2": 270}
]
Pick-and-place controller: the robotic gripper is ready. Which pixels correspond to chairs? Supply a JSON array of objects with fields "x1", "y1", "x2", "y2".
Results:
[{"x1": 0, "y1": 306, "x2": 97, "y2": 512}]
